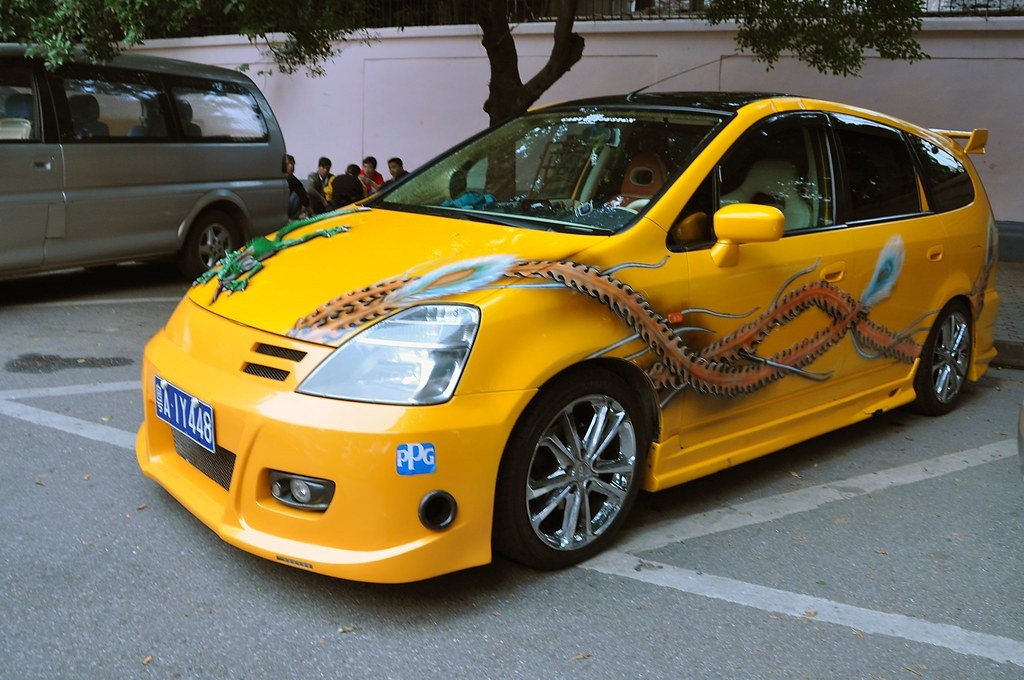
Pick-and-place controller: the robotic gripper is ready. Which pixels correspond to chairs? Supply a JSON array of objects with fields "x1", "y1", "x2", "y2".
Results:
[
  {"x1": 0, "y1": 117, "x2": 32, "y2": 139},
  {"x1": 167, "y1": 98, "x2": 202, "y2": 140},
  {"x1": 68, "y1": 94, "x2": 109, "y2": 141},
  {"x1": 6, "y1": 92, "x2": 35, "y2": 139},
  {"x1": 720, "y1": 148, "x2": 816, "y2": 231},
  {"x1": 127, "y1": 99, "x2": 163, "y2": 138},
  {"x1": 607, "y1": 156, "x2": 667, "y2": 213}
]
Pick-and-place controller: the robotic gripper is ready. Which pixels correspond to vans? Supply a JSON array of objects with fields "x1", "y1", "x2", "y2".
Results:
[{"x1": 0, "y1": 42, "x2": 291, "y2": 285}]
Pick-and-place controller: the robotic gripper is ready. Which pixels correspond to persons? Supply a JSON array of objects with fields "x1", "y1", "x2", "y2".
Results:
[{"x1": 285, "y1": 155, "x2": 408, "y2": 218}]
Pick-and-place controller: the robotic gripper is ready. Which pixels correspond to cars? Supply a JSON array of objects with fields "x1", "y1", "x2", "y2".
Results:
[{"x1": 136, "y1": 83, "x2": 1001, "y2": 584}]
